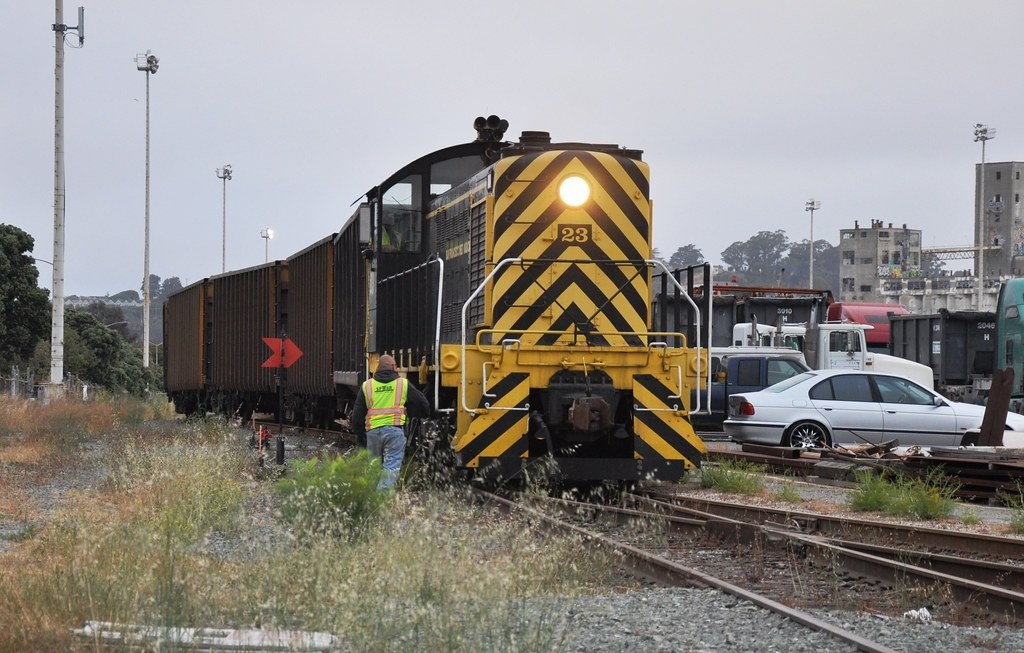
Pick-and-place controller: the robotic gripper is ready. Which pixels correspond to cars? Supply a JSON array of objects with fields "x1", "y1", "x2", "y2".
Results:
[{"x1": 721, "y1": 368, "x2": 1024, "y2": 467}]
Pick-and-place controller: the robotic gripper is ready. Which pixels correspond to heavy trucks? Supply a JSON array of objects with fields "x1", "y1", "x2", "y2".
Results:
[{"x1": 693, "y1": 284, "x2": 912, "y2": 356}]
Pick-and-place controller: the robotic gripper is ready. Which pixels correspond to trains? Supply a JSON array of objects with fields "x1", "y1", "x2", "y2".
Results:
[
  {"x1": 161, "y1": 114, "x2": 711, "y2": 492},
  {"x1": 653, "y1": 289, "x2": 833, "y2": 348}
]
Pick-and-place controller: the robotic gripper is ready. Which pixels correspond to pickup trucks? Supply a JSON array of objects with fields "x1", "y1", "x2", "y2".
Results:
[{"x1": 690, "y1": 349, "x2": 816, "y2": 432}]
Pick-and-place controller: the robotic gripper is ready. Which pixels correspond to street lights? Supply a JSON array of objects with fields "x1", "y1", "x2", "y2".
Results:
[
  {"x1": 215, "y1": 162, "x2": 234, "y2": 276},
  {"x1": 973, "y1": 123, "x2": 997, "y2": 311},
  {"x1": 260, "y1": 228, "x2": 276, "y2": 264},
  {"x1": 805, "y1": 198, "x2": 823, "y2": 290},
  {"x1": 137, "y1": 55, "x2": 161, "y2": 378}
]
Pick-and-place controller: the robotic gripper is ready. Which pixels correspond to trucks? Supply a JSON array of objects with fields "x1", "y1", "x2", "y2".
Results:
[
  {"x1": 708, "y1": 298, "x2": 935, "y2": 394},
  {"x1": 891, "y1": 277, "x2": 1023, "y2": 416}
]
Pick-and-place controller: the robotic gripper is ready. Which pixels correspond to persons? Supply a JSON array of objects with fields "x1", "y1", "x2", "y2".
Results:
[{"x1": 351, "y1": 355, "x2": 430, "y2": 495}]
